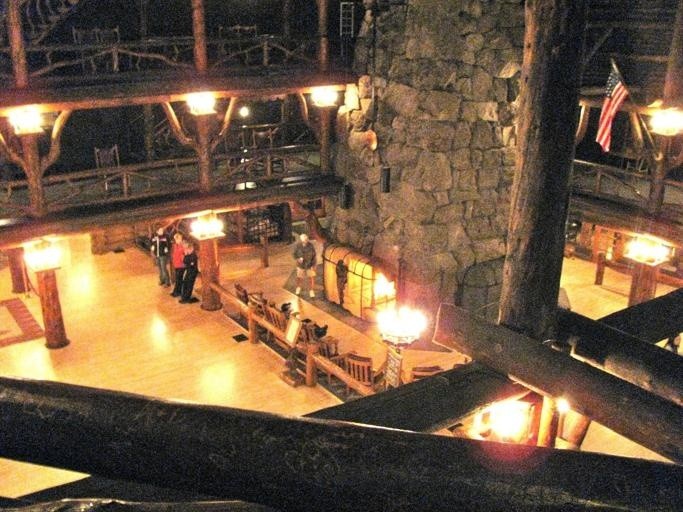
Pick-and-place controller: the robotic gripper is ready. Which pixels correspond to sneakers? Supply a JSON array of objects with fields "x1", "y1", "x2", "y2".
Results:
[
  {"x1": 319, "y1": 325, "x2": 327, "y2": 337},
  {"x1": 171, "y1": 292, "x2": 190, "y2": 304},
  {"x1": 160, "y1": 282, "x2": 171, "y2": 288},
  {"x1": 295, "y1": 287, "x2": 315, "y2": 297}
]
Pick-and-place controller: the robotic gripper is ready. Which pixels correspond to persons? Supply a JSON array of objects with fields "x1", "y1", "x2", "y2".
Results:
[
  {"x1": 292, "y1": 233, "x2": 316, "y2": 296},
  {"x1": 169, "y1": 233, "x2": 186, "y2": 297},
  {"x1": 179, "y1": 243, "x2": 198, "y2": 302},
  {"x1": 149, "y1": 228, "x2": 170, "y2": 286}
]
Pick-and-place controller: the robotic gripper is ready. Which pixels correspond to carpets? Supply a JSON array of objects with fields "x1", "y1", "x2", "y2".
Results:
[{"x1": 0, "y1": 297, "x2": 43, "y2": 349}]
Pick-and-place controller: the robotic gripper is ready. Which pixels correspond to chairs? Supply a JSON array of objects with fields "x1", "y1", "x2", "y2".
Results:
[
  {"x1": 235, "y1": 282, "x2": 386, "y2": 399},
  {"x1": 239, "y1": 124, "x2": 293, "y2": 180},
  {"x1": 95, "y1": 144, "x2": 132, "y2": 196},
  {"x1": 219, "y1": 24, "x2": 262, "y2": 68},
  {"x1": 71, "y1": 24, "x2": 121, "y2": 76}
]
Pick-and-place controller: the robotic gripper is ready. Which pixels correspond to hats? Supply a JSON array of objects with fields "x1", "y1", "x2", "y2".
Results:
[{"x1": 282, "y1": 303, "x2": 291, "y2": 310}]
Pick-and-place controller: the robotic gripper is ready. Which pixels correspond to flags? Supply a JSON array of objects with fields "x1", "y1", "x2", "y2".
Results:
[{"x1": 594, "y1": 65, "x2": 629, "y2": 153}]
